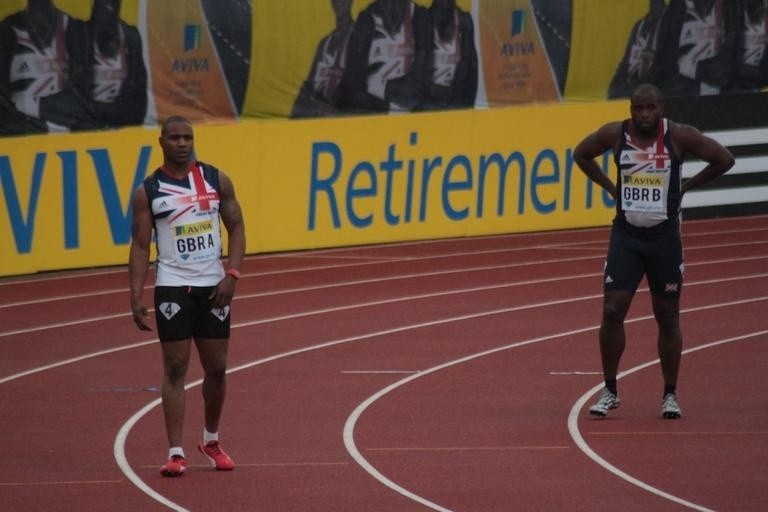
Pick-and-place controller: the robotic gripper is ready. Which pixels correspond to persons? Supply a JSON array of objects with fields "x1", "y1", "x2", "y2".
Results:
[
  {"x1": 413, "y1": 1, "x2": 478, "y2": 114},
  {"x1": 291, "y1": 1, "x2": 357, "y2": 117},
  {"x1": 606, "y1": 0, "x2": 768, "y2": 96},
  {"x1": 72, "y1": 0, "x2": 149, "y2": 134},
  {"x1": 573, "y1": 83, "x2": 734, "y2": 418},
  {"x1": 338, "y1": 1, "x2": 431, "y2": 115},
  {"x1": 0, "y1": 1, "x2": 93, "y2": 139},
  {"x1": 130, "y1": 114, "x2": 246, "y2": 477}
]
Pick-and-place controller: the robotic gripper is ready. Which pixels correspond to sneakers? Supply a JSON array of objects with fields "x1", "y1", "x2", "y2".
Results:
[
  {"x1": 589, "y1": 388, "x2": 620, "y2": 416},
  {"x1": 160, "y1": 454, "x2": 187, "y2": 475},
  {"x1": 198, "y1": 440, "x2": 235, "y2": 470},
  {"x1": 662, "y1": 393, "x2": 682, "y2": 418}
]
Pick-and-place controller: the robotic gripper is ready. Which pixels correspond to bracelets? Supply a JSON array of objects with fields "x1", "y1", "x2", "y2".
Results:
[{"x1": 226, "y1": 268, "x2": 241, "y2": 279}]
